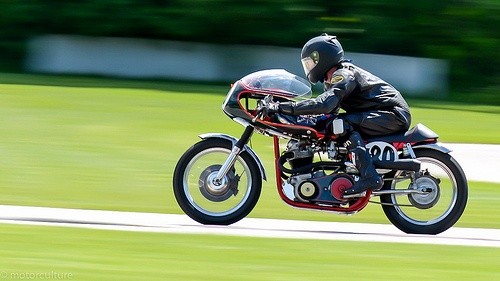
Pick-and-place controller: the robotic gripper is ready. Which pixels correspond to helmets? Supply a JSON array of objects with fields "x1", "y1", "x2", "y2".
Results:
[{"x1": 300, "y1": 33, "x2": 344, "y2": 85}]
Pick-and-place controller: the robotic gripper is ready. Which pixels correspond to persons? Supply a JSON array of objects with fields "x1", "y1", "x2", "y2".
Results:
[{"x1": 263, "y1": 33, "x2": 412, "y2": 197}]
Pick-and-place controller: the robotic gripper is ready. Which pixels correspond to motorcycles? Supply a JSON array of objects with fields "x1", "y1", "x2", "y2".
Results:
[{"x1": 172, "y1": 69, "x2": 468, "y2": 234}]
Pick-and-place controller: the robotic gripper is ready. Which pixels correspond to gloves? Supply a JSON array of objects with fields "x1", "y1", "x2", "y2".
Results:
[{"x1": 257, "y1": 100, "x2": 294, "y2": 116}]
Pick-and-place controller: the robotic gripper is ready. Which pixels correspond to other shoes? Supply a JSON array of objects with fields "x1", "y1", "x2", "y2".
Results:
[{"x1": 343, "y1": 173, "x2": 384, "y2": 195}]
